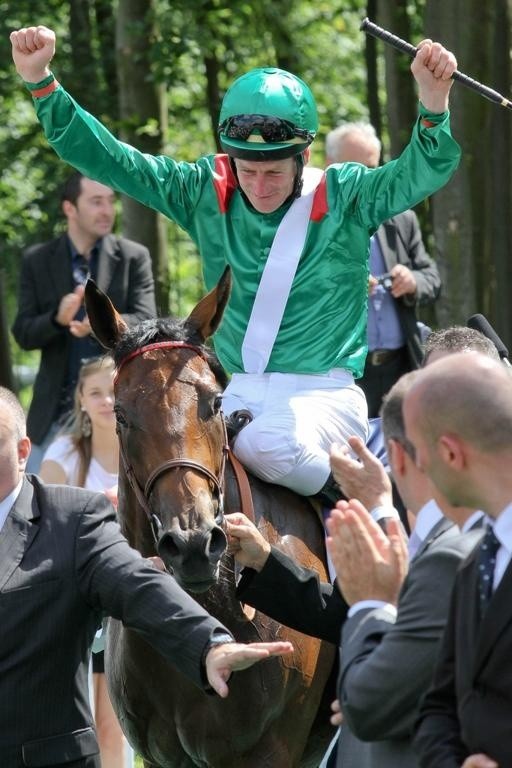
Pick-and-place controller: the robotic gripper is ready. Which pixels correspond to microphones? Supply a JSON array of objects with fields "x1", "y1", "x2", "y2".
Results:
[{"x1": 468, "y1": 314, "x2": 512, "y2": 369}]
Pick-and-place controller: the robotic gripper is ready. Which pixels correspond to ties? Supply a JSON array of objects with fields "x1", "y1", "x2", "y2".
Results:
[{"x1": 474, "y1": 525, "x2": 500, "y2": 618}]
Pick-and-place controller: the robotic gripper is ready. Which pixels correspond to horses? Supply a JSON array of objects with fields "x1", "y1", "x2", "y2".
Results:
[{"x1": 83, "y1": 263, "x2": 412, "y2": 768}]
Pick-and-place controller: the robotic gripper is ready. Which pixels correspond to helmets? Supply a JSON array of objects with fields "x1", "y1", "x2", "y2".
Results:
[{"x1": 217, "y1": 67, "x2": 319, "y2": 162}]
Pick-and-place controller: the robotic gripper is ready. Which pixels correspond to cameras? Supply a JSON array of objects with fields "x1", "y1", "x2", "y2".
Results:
[{"x1": 376, "y1": 272, "x2": 393, "y2": 289}]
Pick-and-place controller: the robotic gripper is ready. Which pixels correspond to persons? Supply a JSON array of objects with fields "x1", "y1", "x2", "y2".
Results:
[
  {"x1": 0, "y1": 389, "x2": 293, "y2": 765},
  {"x1": 213, "y1": 312, "x2": 511, "y2": 768},
  {"x1": 13, "y1": 174, "x2": 158, "y2": 475},
  {"x1": 6, "y1": 26, "x2": 456, "y2": 510},
  {"x1": 322, "y1": 122, "x2": 444, "y2": 533},
  {"x1": 37, "y1": 357, "x2": 135, "y2": 765}
]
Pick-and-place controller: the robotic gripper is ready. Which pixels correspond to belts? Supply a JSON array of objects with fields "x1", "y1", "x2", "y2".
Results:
[{"x1": 363, "y1": 349, "x2": 398, "y2": 365}]
